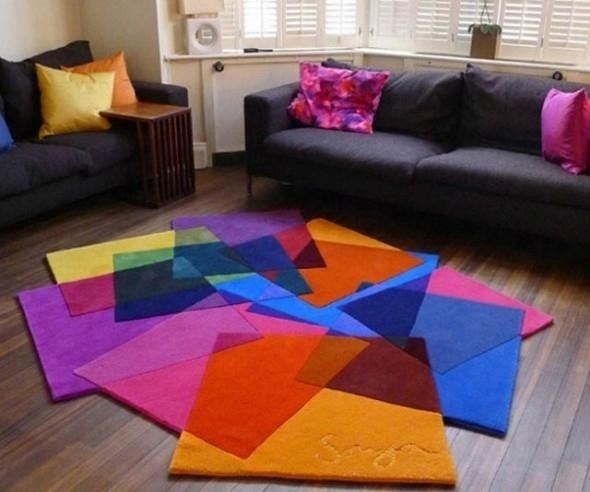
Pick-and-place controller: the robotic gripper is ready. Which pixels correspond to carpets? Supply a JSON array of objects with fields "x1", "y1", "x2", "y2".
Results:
[{"x1": 14, "y1": 206, "x2": 554, "y2": 484}]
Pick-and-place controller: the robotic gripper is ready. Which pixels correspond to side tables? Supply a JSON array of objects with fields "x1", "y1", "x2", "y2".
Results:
[{"x1": 100, "y1": 96, "x2": 197, "y2": 209}]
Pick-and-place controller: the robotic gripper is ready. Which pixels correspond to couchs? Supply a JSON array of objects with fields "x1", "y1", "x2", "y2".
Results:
[
  {"x1": 241, "y1": 59, "x2": 590, "y2": 252},
  {"x1": 1, "y1": 41, "x2": 191, "y2": 230}
]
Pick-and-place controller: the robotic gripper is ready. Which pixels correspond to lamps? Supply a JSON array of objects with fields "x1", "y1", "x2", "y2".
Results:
[{"x1": 176, "y1": 0, "x2": 226, "y2": 55}]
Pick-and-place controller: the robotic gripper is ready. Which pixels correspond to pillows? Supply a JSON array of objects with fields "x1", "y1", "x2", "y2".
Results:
[
  {"x1": 540, "y1": 82, "x2": 590, "y2": 176},
  {"x1": 285, "y1": 59, "x2": 388, "y2": 136},
  {"x1": 0, "y1": 41, "x2": 133, "y2": 150}
]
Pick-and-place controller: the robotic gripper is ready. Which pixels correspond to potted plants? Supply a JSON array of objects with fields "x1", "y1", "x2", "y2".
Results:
[{"x1": 467, "y1": 3, "x2": 500, "y2": 58}]
